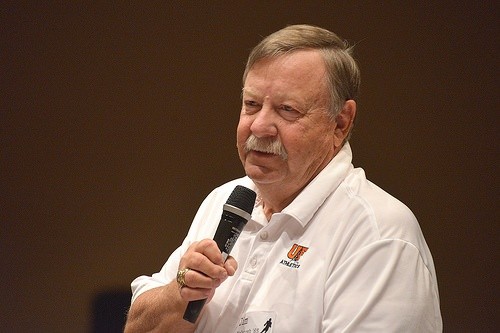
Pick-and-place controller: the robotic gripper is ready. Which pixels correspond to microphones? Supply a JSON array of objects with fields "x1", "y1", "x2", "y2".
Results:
[{"x1": 183, "y1": 185, "x2": 256, "y2": 324}]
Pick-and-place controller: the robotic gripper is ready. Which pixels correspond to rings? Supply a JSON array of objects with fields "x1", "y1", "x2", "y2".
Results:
[{"x1": 177, "y1": 268, "x2": 188, "y2": 285}]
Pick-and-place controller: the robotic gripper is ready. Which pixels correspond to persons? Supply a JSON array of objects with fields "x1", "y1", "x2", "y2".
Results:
[{"x1": 125, "y1": 23, "x2": 443, "y2": 333}]
